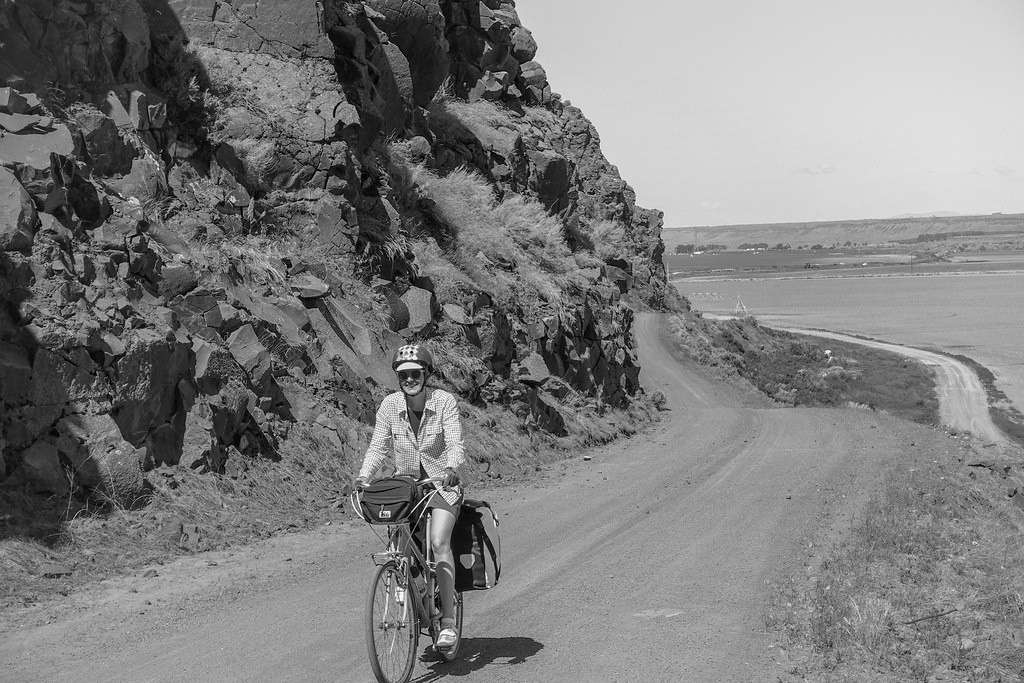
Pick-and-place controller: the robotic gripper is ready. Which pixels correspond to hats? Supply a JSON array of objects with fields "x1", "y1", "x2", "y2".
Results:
[{"x1": 395, "y1": 361, "x2": 427, "y2": 371}]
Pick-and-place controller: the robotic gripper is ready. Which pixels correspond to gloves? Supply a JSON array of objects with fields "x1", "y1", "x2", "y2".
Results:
[
  {"x1": 441, "y1": 468, "x2": 460, "y2": 489},
  {"x1": 352, "y1": 481, "x2": 364, "y2": 497}
]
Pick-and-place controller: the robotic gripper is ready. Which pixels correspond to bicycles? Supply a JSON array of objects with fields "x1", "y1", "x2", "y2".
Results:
[{"x1": 353, "y1": 475, "x2": 465, "y2": 682}]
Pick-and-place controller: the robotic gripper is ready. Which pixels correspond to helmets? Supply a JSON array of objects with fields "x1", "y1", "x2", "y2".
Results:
[{"x1": 392, "y1": 344, "x2": 433, "y2": 374}]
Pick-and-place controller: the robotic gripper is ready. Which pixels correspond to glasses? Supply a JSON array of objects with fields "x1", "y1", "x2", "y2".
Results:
[{"x1": 396, "y1": 370, "x2": 424, "y2": 381}]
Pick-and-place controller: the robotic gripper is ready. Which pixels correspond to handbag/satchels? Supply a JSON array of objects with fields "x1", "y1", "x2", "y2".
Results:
[{"x1": 359, "y1": 477, "x2": 419, "y2": 525}]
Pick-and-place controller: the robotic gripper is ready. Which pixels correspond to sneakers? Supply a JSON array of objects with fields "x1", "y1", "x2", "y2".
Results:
[
  {"x1": 436, "y1": 618, "x2": 460, "y2": 648},
  {"x1": 394, "y1": 581, "x2": 406, "y2": 605}
]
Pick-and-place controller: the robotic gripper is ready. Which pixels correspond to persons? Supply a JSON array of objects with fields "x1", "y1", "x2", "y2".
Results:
[{"x1": 351, "y1": 344, "x2": 471, "y2": 648}]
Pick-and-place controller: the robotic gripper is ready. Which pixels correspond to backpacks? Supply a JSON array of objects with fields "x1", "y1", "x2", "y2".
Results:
[{"x1": 449, "y1": 498, "x2": 503, "y2": 595}]
